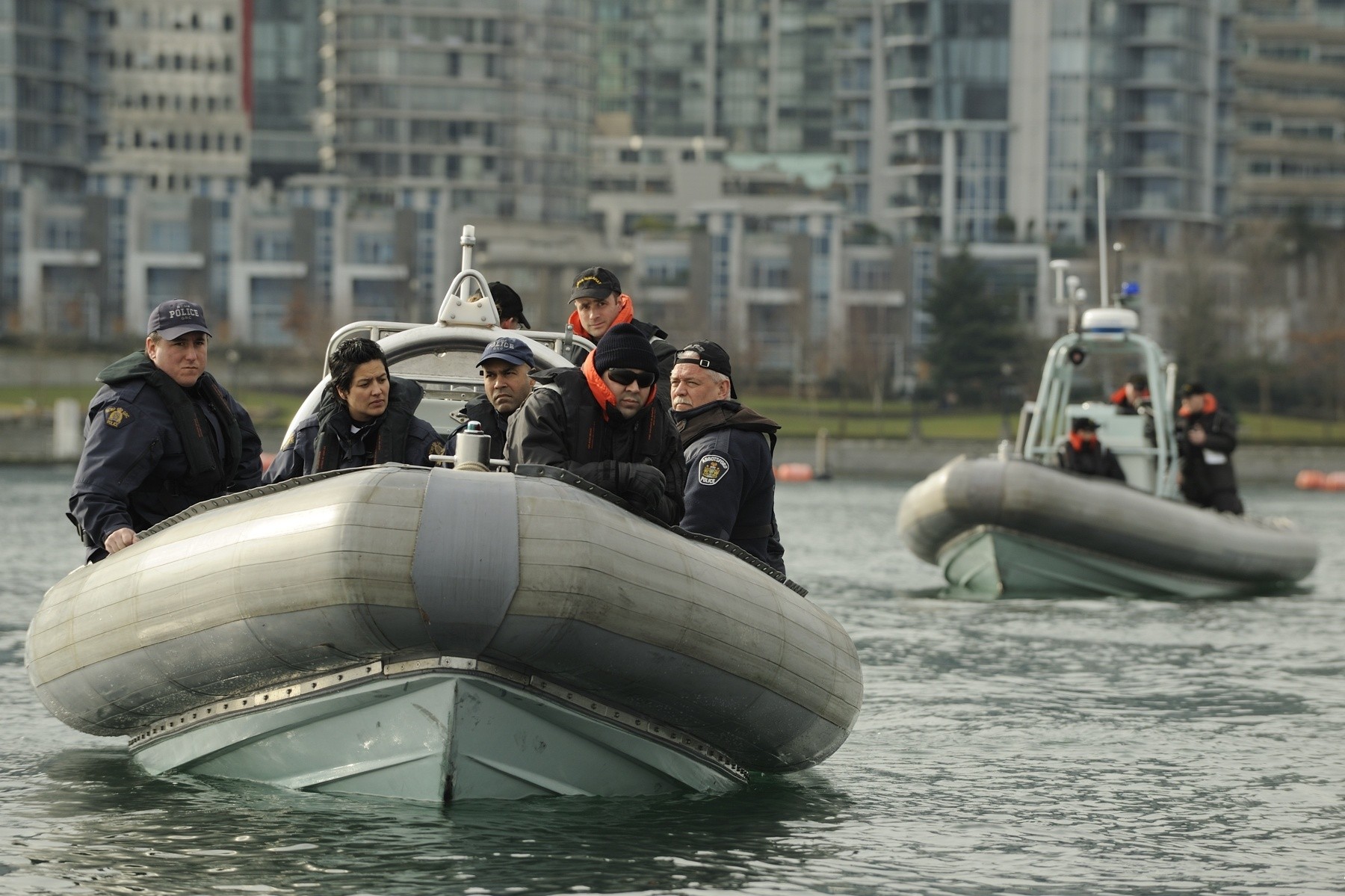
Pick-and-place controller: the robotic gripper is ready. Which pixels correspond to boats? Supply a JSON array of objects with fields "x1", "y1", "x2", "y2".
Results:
[
  {"x1": 894, "y1": 169, "x2": 1321, "y2": 604},
  {"x1": 22, "y1": 221, "x2": 864, "y2": 811}
]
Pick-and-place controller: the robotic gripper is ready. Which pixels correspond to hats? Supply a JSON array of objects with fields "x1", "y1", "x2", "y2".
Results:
[
  {"x1": 593, "y1": 323, "x2": 659, "y2": 386},
  {"x1": 673, "y1": 339, "x2": 737, "y2": 399},
  {"x1": 1128, "y1": 373, "x2": 1148, "y2": 389},
  {"x1": 147, "y1": 299, "x2": 213, "y2": 340},
  {"x1": 567, "y1": 267, "x2": 622, "y2": 304},
  {"x1": 476, "y1": 337, "x2": 535, "y2": 369},
  {"x1": 1177, "y1": 383, "x2": 1206, "y2": 397},
  {"x1": 1072, "y1": 418, "x2": 1101, "y2": 431},
  {"x1": 475, "y1": 281, "x2": 531, "y2": 330}
]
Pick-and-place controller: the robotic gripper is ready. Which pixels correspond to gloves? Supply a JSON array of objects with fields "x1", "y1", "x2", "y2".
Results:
[{"x1": 616, "y1": 460, "x2": 666, "y2": 512}]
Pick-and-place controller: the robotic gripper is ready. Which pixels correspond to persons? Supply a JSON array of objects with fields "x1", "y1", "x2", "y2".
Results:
[
  {"x1": 561, "y1": 267, "x2": 676, "y2": 414},
  {"x1": 495, "y1": 323, "x2": 687, "y2": 529},
  {"x1": 261, "y1": 338, "x2": 447, "y2": 486},
  {"x1": 1174, "y1": 383, "x2": 1243, "y2": 515},
  {"x1": 666, "y1": 339, "x2": 787, "y2": 579},
  {"x1": 1108, "y1": 371, "x2": 1171, "y2": 469},
  {"x1": 66, "y1": 300, "x2": 264, "y2": 564},
  {"x1": 435, "y1": 337, "x2": 538, "y2": 473},
  {"x1": 1057, "y1": 417, "x2": 1125, "y2": 480},
  {"x1": 446, "y1": 281, "x2": 530, "y2": 333}
]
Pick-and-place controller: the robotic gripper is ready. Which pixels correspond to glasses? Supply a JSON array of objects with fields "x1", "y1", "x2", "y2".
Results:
[
  {"x1": 606, "y1": 368, "x2": 655, "y2": 388},
  {"x1": 510, "y1": 318, "x2": 523, "y2": 331}
]
